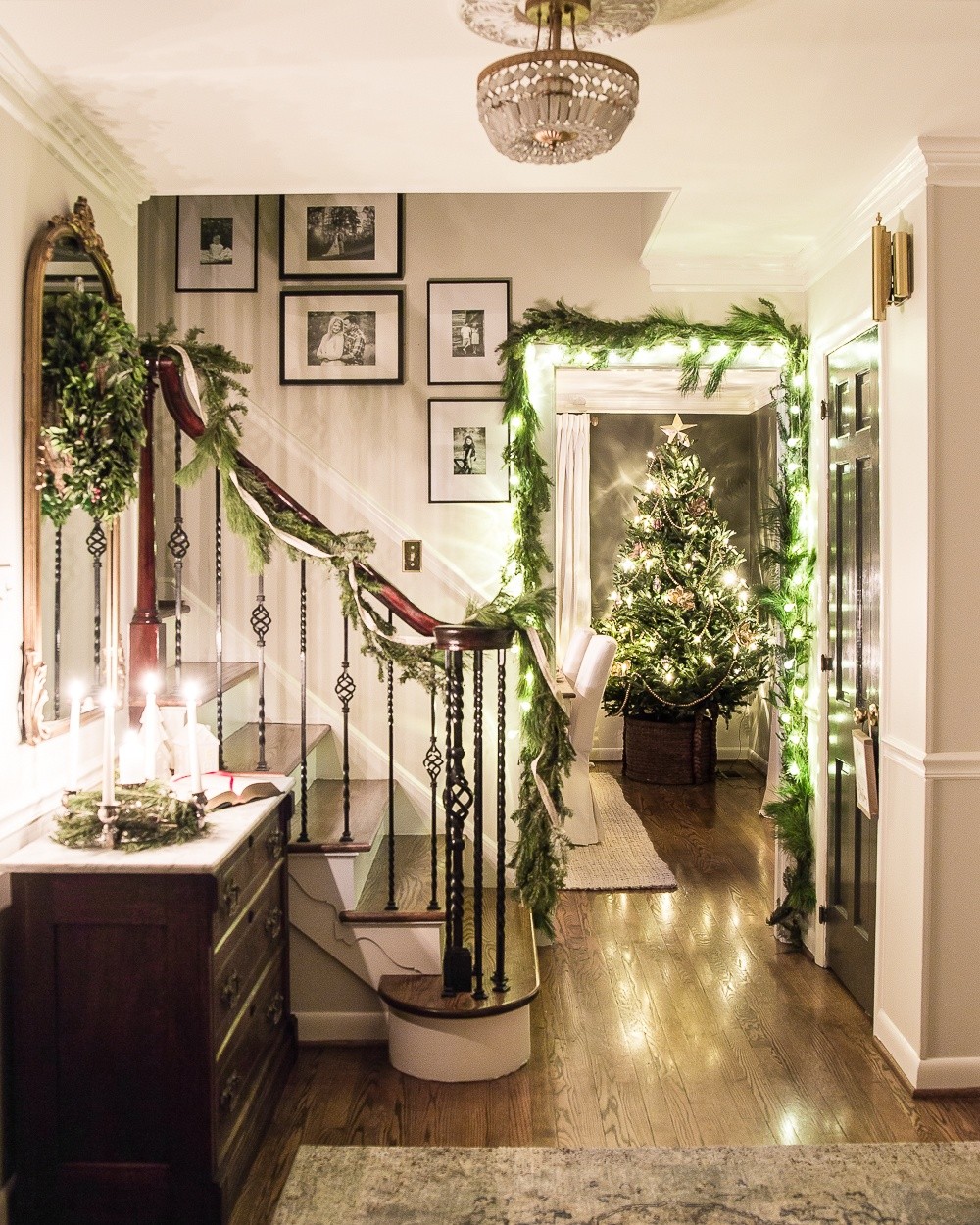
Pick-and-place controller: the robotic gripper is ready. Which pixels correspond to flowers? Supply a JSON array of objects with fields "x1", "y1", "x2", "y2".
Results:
[{"x1": 31, "y1": 289, "x2": 151, "y2": 531}]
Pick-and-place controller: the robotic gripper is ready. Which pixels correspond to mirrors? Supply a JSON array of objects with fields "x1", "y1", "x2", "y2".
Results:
[{"x1": 22, "y1": 192, "x2": 128, "y2": 745}]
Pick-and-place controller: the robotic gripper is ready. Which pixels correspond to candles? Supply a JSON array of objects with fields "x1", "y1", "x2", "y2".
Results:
[
  {"x1": 140, "y1": 672, "x2": 165, "y2": 770},
  {"x1": 182, "y1": 683, "x2": 202, "y2": 796},
  {"x1": 65, "y1": 677, "x2": 89, "y2": 789},
  {"x1": 99, "y1": 685, "x2": 118, "y2": 801}
]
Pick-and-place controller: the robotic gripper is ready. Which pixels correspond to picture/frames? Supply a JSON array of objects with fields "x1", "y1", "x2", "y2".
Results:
[
  {"x1": 428, "y1": 279, "x2": 511, "y2": 386},
  {"x1": 176, "y1": 195, "x2": 258, "y2": 292},
  {"x1": 279, "y1": 289, "x2": 405, "y2": 385},
  {"x1": 427, "y1": 397, "x2": 511, "y2": 503},
  {"x1": 279, "y1": 194, "x2": 404, "y2": 278}
]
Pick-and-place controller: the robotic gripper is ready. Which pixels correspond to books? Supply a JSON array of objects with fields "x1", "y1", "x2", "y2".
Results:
[{"x1": 167, "y1": 771, "x2": 282, "y2": 813}]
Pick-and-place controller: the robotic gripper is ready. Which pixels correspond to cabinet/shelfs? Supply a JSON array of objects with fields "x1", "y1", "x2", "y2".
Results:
[{"x1": 9, "y1": 796, "x2": 301, "y2": 1221}]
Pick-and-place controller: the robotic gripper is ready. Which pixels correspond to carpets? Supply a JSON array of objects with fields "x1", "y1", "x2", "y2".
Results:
[
  {"x1": 550, "y1": 771, "x2": 678, "y2": 890},
  {"x1": 270, "y1": 1141, "x2": 979, "y2": 1225}
]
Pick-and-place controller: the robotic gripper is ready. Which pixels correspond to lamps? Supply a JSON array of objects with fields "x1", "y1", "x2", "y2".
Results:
[
  {"x1": 462, "y1": 0, "x2": 657, "y2": 163},
  {"x1": 871, "y1": 210, "x2": 909, "y2": 320}
]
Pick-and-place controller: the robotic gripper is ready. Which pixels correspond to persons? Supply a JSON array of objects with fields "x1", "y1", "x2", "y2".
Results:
[
  {"x1": 322, "y1": 230, "x2": 344, "y2": 256},
  {"x1": 460, "y1": 322, "x2": 479, "y2": 354},
  {"x1": 462, "y1": 436, "x2": 476, "y2": 474},
  {"x1": 316, "y1": 316, "x2": 365, "y2": 365},
  {"x1": 208, "y1": 235, "x2": 223, "y2": 258}
]
